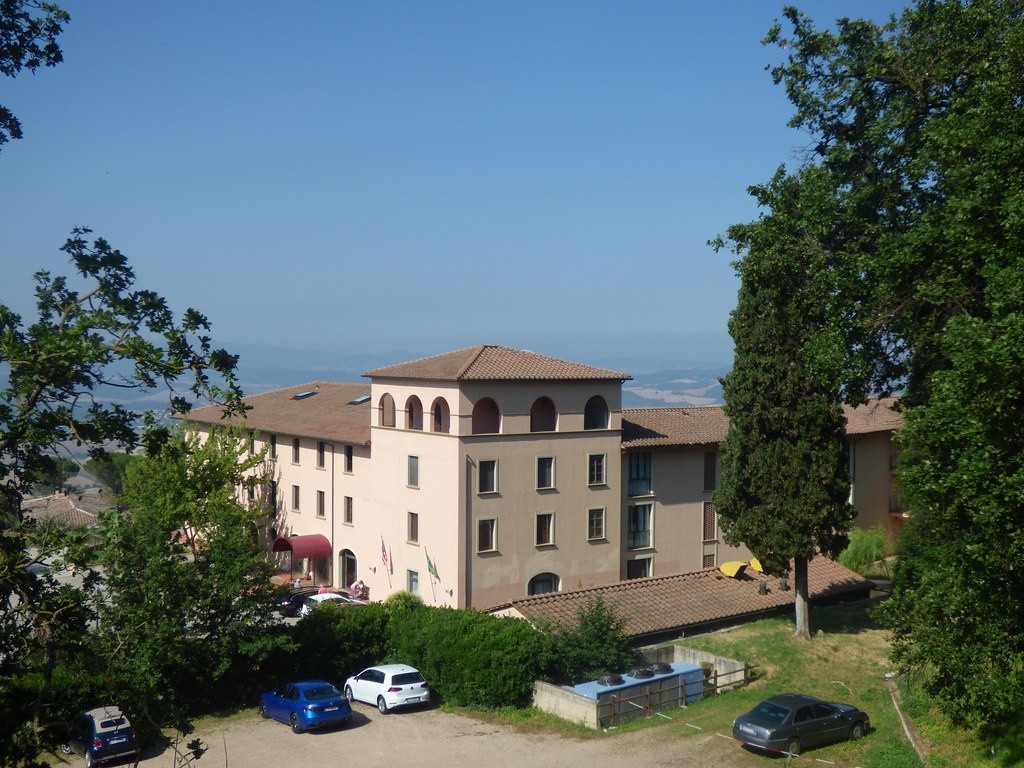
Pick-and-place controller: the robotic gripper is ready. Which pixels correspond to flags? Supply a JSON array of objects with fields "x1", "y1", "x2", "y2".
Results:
[
  {"x1": 381, "y1": 542, "x2": 387, "y2": 565},
  {"x1": 425, "y1": 550, "x2": 439, "y2": 581}
]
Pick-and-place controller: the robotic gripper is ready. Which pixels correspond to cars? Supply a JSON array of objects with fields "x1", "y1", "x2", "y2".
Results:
[
  {"x1": 733, "y1": 693, "x2": 871, "y2": 758},
  {"x1": 258, "y1": 678, "x2": 352, "y2": 734},
  {"x1": 299, "y1": 592, "x2": 367, "y2": 617},
  {"x1": 343, "y1": 663, "x2": 431, "y2": 716},
  {"x1": 277, "y1": 586, "x2": 353, "y2": 618},
  {"x1": 58, "y1": 704, "x2": 143, "y2": 768}
]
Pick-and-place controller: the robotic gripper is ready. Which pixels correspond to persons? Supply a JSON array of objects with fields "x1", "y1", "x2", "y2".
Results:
[
  {"x1": 318, "y1": 584, "x2": 328, "y2": 594},
  {"x1": 350, "y1": 580, "x2": 363, "y2": 599}
]
